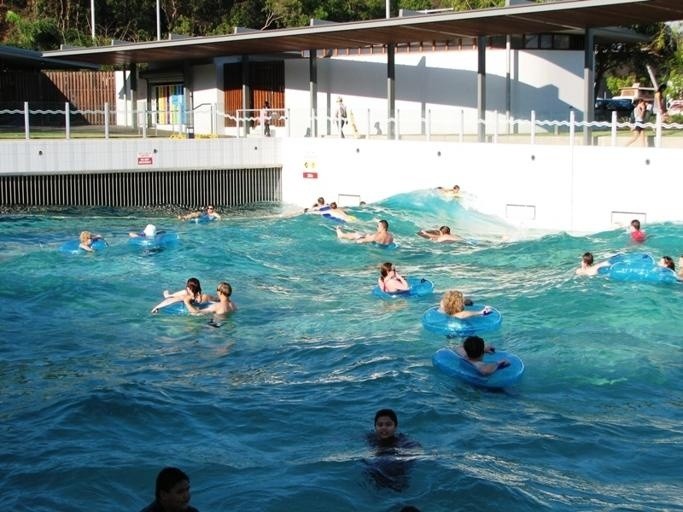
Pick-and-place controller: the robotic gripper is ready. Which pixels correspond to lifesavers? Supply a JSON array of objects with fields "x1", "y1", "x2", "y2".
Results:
[
  {"x1": 128, "y1": 229, "x2": 180, "y2": 247},
  {"x1": 371, "y1": 278, "x2": 435, "y2": 300},
  {"x1": 610, "y1": 261, "x2": 677, "y2": 287},
  {"x1": 162, "y1": 301, "x2": 213, "y2": 316},
  {"x1": 368, "y1": 242, "x2": 399, "y2": 250},
  {"x1": 422, "y1": 304, "x2": 500, "y2": 336},
  {"x1": 598, "y1": 254, "x2": 653, "y2": 272},
  {"x1": 189, "y1": 215, "x2": 210, "y2": 224},
  {"x1": 431, "y1": 346, "x2": 524, "y2": 390},
  {"x1": 58, "y1": 237, "x2": 109, "y2": 254}
]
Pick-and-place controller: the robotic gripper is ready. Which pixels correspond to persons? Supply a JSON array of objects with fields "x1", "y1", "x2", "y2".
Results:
[
  {"x1": 335, "y1": 97, "x2": 348, "y2": 138},
  {"x1": 312, "y1": 202, "x2": 358, "y2": 225},
  {"x1": 142, "y1": 468, "x2": 200, "y2": 511},
  {"x1": 183, "y1": 281, "x2": 237, "y2": 327},
  {"x1": 455, "y1": 335, "x2": 511, "y2": 376},
  {"x1": 658, "y1": 255, "x2": 675, "y2": 272},
  {"x1": 129, "y1": 224, "x2": 157, "y2": 242},
  {"x1": 575, "y1": 252, "x2": 611, "y2": 276},
  {"x1": 78, "y1": 230, "x2": 102, "y2": 253},
  {"x1": 374, "y1": 409, "x2": 398, "y2": 440},
  {"x1": 439, "y1": 289, "x2": 494, "y2": 320},
  {"x1": 336, "y1": 219, "x2": 394, "y2": 247},
  {"x1": 151, "y1": 278, "x2": 221, "y2": 316},
  {"x1": 436, "y1": 184, "x2": 460, "y2": 196},
  {"x1": 420, "y1": 226, "x2": 459, "y2": 244},
  {"x1": 676, "y1": 256, "x2": 683, "y2": 279},
  {"x1": 627, "y1": 87, "x2": 683, "y2": 126},
  {"x1": 311, "y1": 196, "x2": 330, "y2": 212},
  {"x1": 377, "y1": 262, "x2": 410, "y2": 294},
  {"x1": 178, "y1": 204, "x2": 222, "y2": 226},
  {"x1": 630, "y1": 219, "x2": 645, "y2": 242},
  {"x1": 263, "y1": 101, "x2": 272, "y2": 137},
  {"x1": 624, "y1": 98, "x2": 646, "y2": 147},
  {"x1": 303, "y1": 207, "x2": 311, "y2": 215}
]
